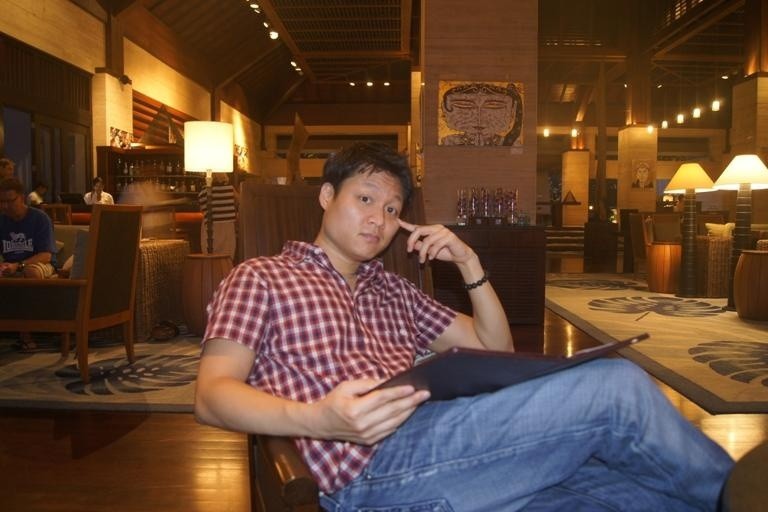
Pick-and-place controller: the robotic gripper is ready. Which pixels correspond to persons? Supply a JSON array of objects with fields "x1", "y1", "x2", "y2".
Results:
[
  {"x1": 194, "y1": 144, "x2": 734, "y2": 512},
  {"x1": 26, "y1": 182, "x2": 48, "y2": 205},
  {"x1": 84, "y1": 177, "x2": 114, "y2": 207},
  {"x1": 633, "y1": 163, "x2": 655, "y2": 187},
  {"x1": 0, "y1": 179, "x2": 58, "y2": 351},
  {"x1": 197, "y1": 170, "x2": 239, "y2": 260},
  {"x1": 0, "y1": 158, "x2": 14, "y2": 176}
]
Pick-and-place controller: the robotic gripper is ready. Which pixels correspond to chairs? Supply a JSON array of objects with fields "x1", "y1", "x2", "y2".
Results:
[
  {"x1": 0, "y1": 195, "x2": 197, "y2": 389},
  {"x1": 239, "y1": 410, "x2": 325, "y2": 512},
  {"x1": 620, "y1": 204, "x2": 768, "y2": 301}
]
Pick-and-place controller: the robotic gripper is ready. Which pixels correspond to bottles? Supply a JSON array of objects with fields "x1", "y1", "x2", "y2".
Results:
[
  {"x1": 114, "y1": 152, "x2": 204, "y2": 192},
  {"x1": 455, "y1": 185, "x2": 530, "y2": 227}
]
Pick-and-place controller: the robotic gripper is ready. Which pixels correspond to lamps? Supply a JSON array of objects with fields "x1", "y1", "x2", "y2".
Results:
[
  {"x1": 178, "y1": 119, "x2": 239, "y2": 255},
  {"x1": 346, "y1": 68, "x2": 393, "y2": 89},
  {"x1": 705, "y1": 153, "x2": 768, "y2": 312},
  {"x1": 243, "y1": 1, "x2": 303, "y2": 76},
  {"x1": 663, "y1": 160, "x2": 720, "y2": 300},
  {"x1": 635, "y1": 24, "x2": 722, "y2": 136}
]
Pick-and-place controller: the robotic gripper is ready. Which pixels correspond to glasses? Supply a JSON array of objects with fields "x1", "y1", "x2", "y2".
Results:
[{"x1": 0, "y1": 196, "x2": 20, "y2": 204}]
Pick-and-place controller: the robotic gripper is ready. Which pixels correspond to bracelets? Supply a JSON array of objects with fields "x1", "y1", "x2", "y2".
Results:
[{"x1": 464, "y1": 274, "x2": 488, "y2": 289}]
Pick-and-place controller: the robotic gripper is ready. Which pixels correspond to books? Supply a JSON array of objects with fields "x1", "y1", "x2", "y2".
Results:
[{"x1": 363, "y1": 330, "x2": 649, "y2": 401}]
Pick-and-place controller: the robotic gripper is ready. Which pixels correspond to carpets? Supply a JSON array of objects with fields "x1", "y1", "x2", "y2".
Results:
[
  {"x1": 538, "y1": 266, "x2": 767, "y2": 420},
  {"x1": 1, "y1": 331, "x2": 208, "y2": 419}
]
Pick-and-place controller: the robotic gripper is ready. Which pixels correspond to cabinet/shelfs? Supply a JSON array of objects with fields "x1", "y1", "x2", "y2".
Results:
[
  {"x1": 417, "y1": 217, "x2": 549, "y2": 328},
  {"x1": 93, "y1": 139, "x2": 239, "y2": 213}
]
